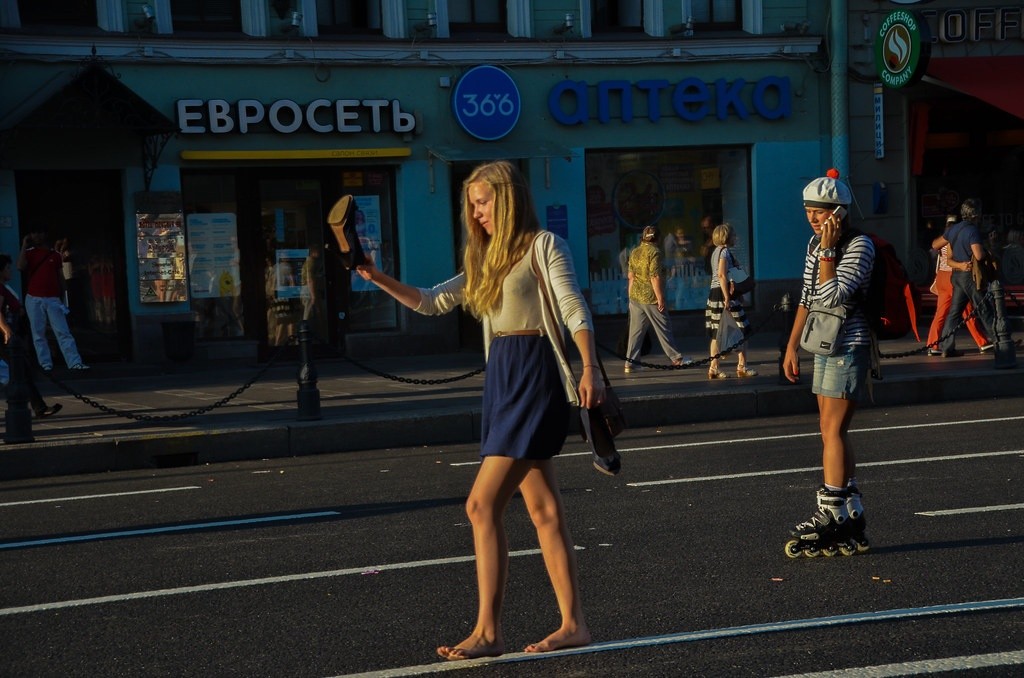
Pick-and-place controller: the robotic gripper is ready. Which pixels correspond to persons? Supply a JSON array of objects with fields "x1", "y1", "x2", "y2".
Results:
[
  {"x1": 706, "y1": 223, "x2": 758, "y2": 378},
  {"x1": 782, "y1": 168, "x2": 875, "y2": 558},
  {"x1": 357, "y1": 162, "x2": 606, "y2": 659},
  {"x1": 16, "y1": 228, "x2": 90, "y2": 372},
  {"x1": 189, "y1": 244, "x2": 322, "y2": 343},
  {"x1": 0, "y1": 255, "x2": 63, "y2": 418},
  {"x1": 619, "y1": 215, "x2": 718, "y2": 279},
  {"x1": 624, "y1": 224, "x2": 692, "y2": 373},
  {"x1": 925, "y1": 199, "x2": 1024, "y2": 357}
]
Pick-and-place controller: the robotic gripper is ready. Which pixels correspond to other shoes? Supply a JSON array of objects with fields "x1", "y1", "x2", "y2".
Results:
[
  {"x1": 928, "y1": 348, "x2": 942, "y2": 357},
  {"x1": 941, "y1": 349, "x2": 965, "y2": 357},
  {"x1": 40, "y1": 403, "x2": 63, "y2": 419},
  {"x1": 978, "y1": 344, "x2": 995, "y2": 354},
  {"x1": 72, "y1": 363, "x2": 90, "y2": 369},
  {"x1": 44, "y1": 366, "x2": 53, "y2": 370}
]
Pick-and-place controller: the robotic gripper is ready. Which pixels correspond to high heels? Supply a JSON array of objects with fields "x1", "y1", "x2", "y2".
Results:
[
  {"x1": 708, "y1": 366, "x2": 731, "y2": 379},
  {"x1": 579, "y1": 408, "x2": 621, "y2": 478},
  {"x1": 326, "y1": 195, "x2": 367, "y2": 283},
  {"x1": 624, "y1": 363, "x2": 645, "y2": 373},
  {"x1": 736, "y1": 364, "x2": 758, "y2": 378},
  {"x1": 673, "y1": 357, "x2": 694, "y2": 366}
]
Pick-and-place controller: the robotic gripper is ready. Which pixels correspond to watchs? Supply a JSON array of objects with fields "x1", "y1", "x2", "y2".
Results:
[{"x1": 820, "y1": 249, "x2": 835, "y2": 258}]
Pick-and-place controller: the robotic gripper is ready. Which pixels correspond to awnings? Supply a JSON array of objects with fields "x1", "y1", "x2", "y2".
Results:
[
  {"x1": 0, "y1": 62, "x2": 183, "y2": 191},
  {"x1": 926, "y1": 55, "x2": 1024, "y2": 121}
]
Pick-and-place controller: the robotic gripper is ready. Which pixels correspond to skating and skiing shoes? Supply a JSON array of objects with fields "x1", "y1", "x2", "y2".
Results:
[
  {"x1": 785, "y1": 485, "x2": 858, "y2": 557},
  {"x1": 844, "y1": 483, "x2": 871, "y2": 553}
]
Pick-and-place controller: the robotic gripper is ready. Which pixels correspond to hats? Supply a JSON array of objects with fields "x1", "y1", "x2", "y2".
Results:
[
  {"x1": 946, "y1": 214, "x2": 958, "y2": 222},
  {"x1": 802, "y1": 168, "x2": 852, "y2": 211}
]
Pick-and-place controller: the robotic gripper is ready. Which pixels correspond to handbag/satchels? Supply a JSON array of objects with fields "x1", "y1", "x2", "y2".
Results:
[
  {"x1": 716, "y1": 308, "x2": 746, "y2": 360},
  {"x1": 718, "y1": 247, "x2": 756, "y2": 298},
  {"x1": 580, "y1": 385, "x2": 625, "y2": 441},
  {"x1": 930, "y1": 274, "x2": 938, "y2": 295},
  {"x1": 799, "y1": 299, "x2": 851, "y2": 356},
  {"x1": 61, "y1": 256, "x2": 73, "y2": 280}
]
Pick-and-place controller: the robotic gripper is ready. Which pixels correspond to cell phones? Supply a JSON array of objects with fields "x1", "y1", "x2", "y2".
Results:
[{"x1": 821, "y1": 206, "x2": 848, "y2": 231}]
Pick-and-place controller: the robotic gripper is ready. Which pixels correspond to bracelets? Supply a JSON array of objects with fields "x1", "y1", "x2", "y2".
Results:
[
  {"x1": 820, "y1": 258, "x2": 834, "y2": 261},
  {"x1": 584, "y1": 365, "x2": 600, "y2": 369}
]
